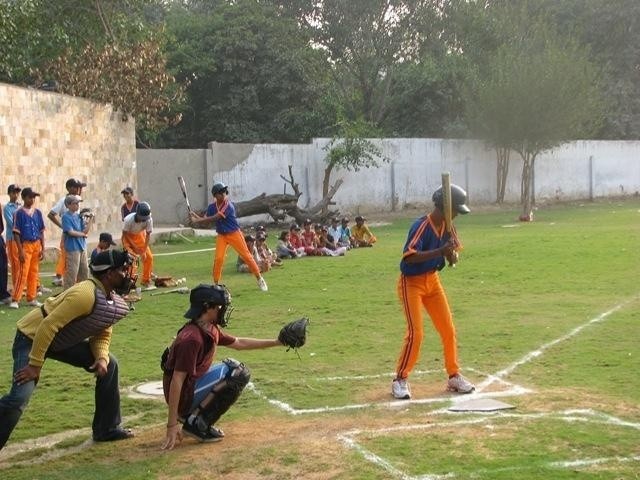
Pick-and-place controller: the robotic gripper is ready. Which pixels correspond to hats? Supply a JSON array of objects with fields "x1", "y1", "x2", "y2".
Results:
[
  {"x1": 246, "y1": 225, "x2": 268, "y2": 241},
  {"x1": 65, "y1": 195, "x2": 83, "y2": 208},
  {"x1": 121, "y1": 187, "x2": 133, "y2": 193},
  {"x1": 292, "y1": 219, "x2": 328, "y2": 231},
  {"x1": 66, "y1": 178, "x2": 86, "y2": 188},
  {"x1": 22, "y1": 188, "x2": 40, "y2": 196},
  {"x1": 8, "y1": 184, "x2": 21, "y2": 194},
  {"x1": 332, "y1": 215, "x2": 366, "y2": 222}
]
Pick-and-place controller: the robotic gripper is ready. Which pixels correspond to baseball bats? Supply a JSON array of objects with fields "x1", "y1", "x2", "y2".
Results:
[
  {"x1": 151, "y1": 287, "x2": 178, "y2": 297},
  {"x1": 176, "y1": 176, "x2": 191, "y2": 217},
  {"x1": 442, "y1": 173, "x2": 456, "y2": 268},
  {"x1": 130, "y1": 286, "x2": 157, "y2": 293}
]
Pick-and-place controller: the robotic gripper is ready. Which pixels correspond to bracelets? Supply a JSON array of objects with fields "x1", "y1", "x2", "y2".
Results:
[{"x1": 166, "y1": 422, "x2": 179, "y2": 428}]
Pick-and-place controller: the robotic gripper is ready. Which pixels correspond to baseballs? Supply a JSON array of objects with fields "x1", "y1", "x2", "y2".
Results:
[{"x1": 178, "y1": 278, "x2": 186, "y2": 284}]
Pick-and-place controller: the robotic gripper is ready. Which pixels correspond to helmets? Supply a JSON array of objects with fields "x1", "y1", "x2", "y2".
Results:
[
  {"x1": 212, "y1": 183, "x2": 228, "y2": 196},
  {"x1": 184, "y1": 284, "x2": 233, "y2": 327},
  {"x1": 90, "y1": 249, "x2": 139, "y2": 294},
  {"x1": 432, "y1": 183, "x2": 471, "y2": 213},
  {"x1": 137, "y1": 202, "x2": 151, "y2": 220}
]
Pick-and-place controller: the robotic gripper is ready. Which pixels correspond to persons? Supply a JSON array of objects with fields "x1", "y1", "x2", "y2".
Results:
[
  {"x1": 392, "y1": 184, "x2": 475, "y2": 400},
  {"x1": 0, "y1": 206, "x2": 12, "y2": 304},
  {"x1": 190, "y1": 183, "x2": 268, "y2": 292},
  {"x1": 91, "y1": 234, "x2": 116, "y2": 263},
  {"x1": 237, "y1": 216, "x2": 377, "y2": 274},
  {"x1": 121, "y1": 187, "x2": 140, "y2": 268},
  {"x1": 121, "y1": 202, "x2": 154, "y2": 287},
  {"x1": 0, "y1": 250, "x2": 138, "y2": 454},
  {"x1": 61, "y1": 196, "x2": 90, "y2": 291},
  {"x1": 159, "y1": 284, "x2": 310, "y2": 451},
  {"x1": 3, "y1": 183, "x2": 52, "y2": 296},
  {"x1": 9, "y1": 188, "x2": 46, "y2": 309},
  {"x1": 47, "y1": 177, "x2": 87, "y2": 286}
]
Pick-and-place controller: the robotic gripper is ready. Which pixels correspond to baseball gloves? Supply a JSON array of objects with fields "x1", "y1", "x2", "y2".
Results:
[{"x1": 279, "y1": 318, "x2": 309, "y2": 347}]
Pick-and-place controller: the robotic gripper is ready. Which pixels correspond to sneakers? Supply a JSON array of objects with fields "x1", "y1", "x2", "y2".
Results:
[
  {"x1": 3, "y1": 286, "x2": 52, "y2": 308},
  {"x1": 52, "y1": 279, "x2": 63, "y2": 286},
  {"x1": 258, "y1": 277, "x2": 268, "y2": 292},
  {"x1": 181, "y1": 415, "x2": 223, "y2": 443},
  {"x1": 447, "y1": 374, "x2": 475, "y2": 394},
  {"x1": 391, "y1": 379, "x2": 411, "y2": 398},
  {"x1": 93, "y1": 426, "x2": 134, "y2": 442}
]
